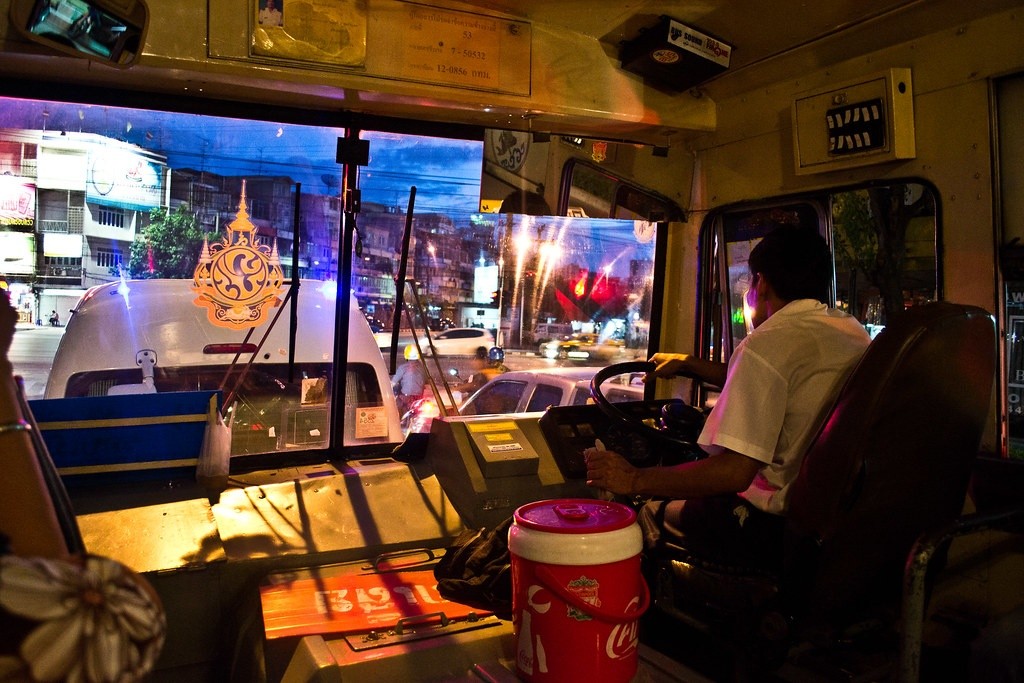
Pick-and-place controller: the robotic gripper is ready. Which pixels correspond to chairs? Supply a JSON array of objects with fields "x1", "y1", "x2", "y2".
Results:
[{"x1": 651, "y1": 299, "x2": 1000, "y2": 646}]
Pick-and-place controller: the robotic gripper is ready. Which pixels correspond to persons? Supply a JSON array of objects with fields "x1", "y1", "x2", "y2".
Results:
[
  {"x1": 584, "y1": 222, "x2": 872, "y2": 561},
  {"x1": 259, "y1": 0, "x2": 283, "y2": 26},
  {"x1": 0, "y1": 287, "x2": 69, "y2": 564},
  {"x1": 390, "y1": 345, "x2": 427, "y2": 421},
  {"x1": 488, "y1": 347, "x2": 511, "y2": 373},
  {"x1": 48, "y1": 310, "x2": 58, "y2": 326},
  {"x1": 499, "y1": 190, "x2": 553, "y2": 215},
  {"x1": 453, "y1": 346, "x2": 505, "y2": 414}
]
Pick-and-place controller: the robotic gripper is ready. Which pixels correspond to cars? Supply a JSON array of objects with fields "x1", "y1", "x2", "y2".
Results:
[
  {"x1": 226, "y1": 399, "x2": 298, "y2": 444},
  {"x1": 526, "y1": 325, "x2": 648, "y2": 365}
]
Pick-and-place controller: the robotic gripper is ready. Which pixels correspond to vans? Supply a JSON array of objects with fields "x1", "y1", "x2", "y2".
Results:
[
  {"x1": 452, "y1": 366, "x2": 646, "y2": 418},
  {"x1": 413, "y1": 327, "x2": 495, "y2": 359},
  {"x1": 43, "y1": 277, "x2": 404, "y2": 458}
]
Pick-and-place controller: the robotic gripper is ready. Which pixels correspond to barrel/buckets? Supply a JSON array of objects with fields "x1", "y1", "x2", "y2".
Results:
[{"x1": 506, "y1": 498, "x2": 652, "y2": 683}]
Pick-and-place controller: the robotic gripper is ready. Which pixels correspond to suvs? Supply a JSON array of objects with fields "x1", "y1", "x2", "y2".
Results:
[
  {"x1": 366, "y1": 318, "x2": 385, "y2": 332},
  {"x1": 428, "y1": 317, "x2": 456, "y2": 331}
]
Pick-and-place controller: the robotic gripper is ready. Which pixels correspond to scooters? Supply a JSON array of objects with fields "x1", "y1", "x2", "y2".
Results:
[{"x1": 390, "y1": 379, "x2": 477, "y2": 441}]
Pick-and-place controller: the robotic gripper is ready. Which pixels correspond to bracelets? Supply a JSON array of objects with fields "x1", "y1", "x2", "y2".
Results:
[{"x1": 0, "y1": 423, "x2": 32, "y2": 433}]
